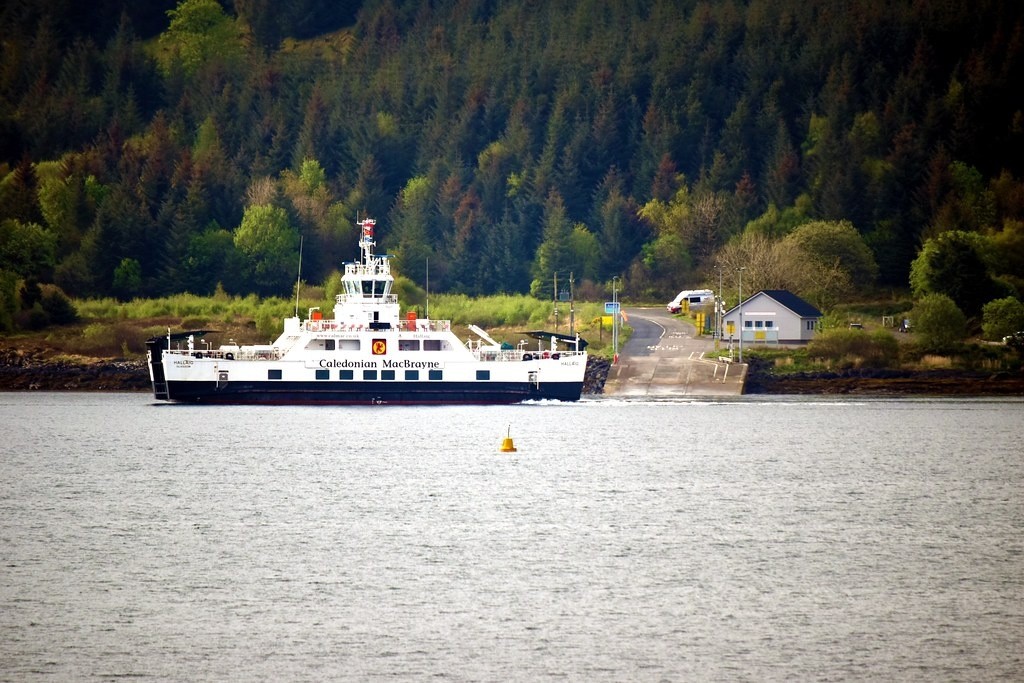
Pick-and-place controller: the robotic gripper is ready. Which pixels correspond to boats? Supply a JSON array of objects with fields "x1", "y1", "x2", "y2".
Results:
[{"x1": 146, "y1": 207, "x2": 590, "y2": 404}]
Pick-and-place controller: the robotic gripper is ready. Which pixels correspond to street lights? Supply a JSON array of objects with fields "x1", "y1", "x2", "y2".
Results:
[
  {"x1": 714, "y1": 266, "x2": 726, "y2": 340},
  {"x1": 612, "y1": 276, "x2": 621, "y2": 353},
  {"x1": 736, "y1": 267, "x2": 748, "y2": 363}
]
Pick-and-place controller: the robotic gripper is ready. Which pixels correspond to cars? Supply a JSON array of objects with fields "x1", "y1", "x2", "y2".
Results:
[
  {"x1": 899, "y1": 319, "x2": 916, "y2": 333},
  {"x1": 1002, "y1": 331, "x2": 1024, "y2": 341},
  {"x1": 848, "y1": 323, "x2": 864, "y2": 331}
]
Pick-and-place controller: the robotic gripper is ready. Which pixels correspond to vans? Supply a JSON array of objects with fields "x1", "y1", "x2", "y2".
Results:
[{"x1": 667, "y1": 289, "x2": 714, "y2": 314}]
